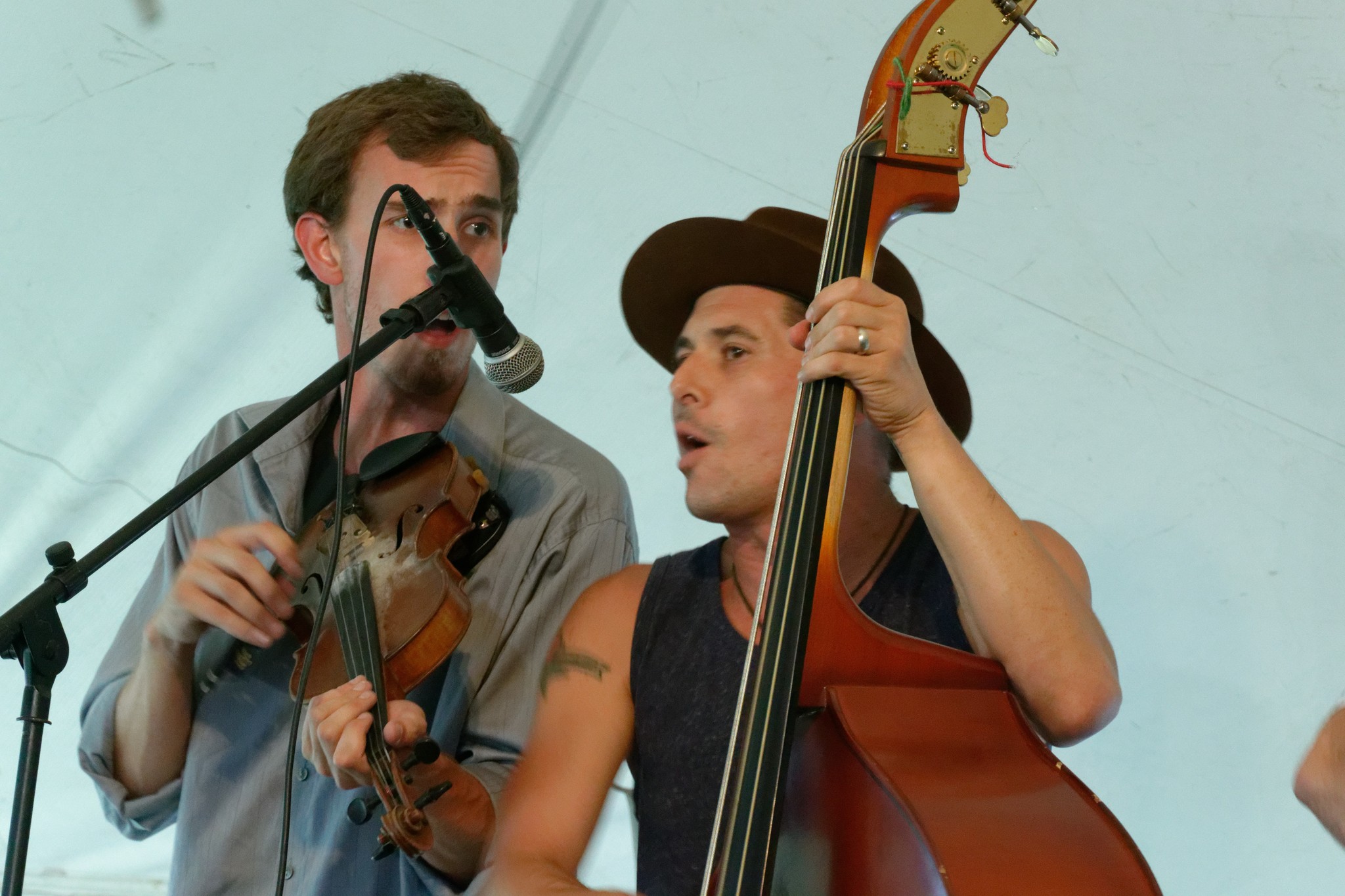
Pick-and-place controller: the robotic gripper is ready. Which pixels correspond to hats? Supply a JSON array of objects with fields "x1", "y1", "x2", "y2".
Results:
[{"x1": 619, "y1": 206, "x2": 973, "y2": 473}]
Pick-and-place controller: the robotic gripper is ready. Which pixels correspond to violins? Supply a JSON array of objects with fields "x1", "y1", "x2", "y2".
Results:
[{"x1": 267, "y1": 431, "x2": 514, "y2": 878}]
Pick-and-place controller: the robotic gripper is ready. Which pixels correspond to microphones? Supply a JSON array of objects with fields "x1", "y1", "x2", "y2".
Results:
[{"x1": 399, "y1": 186, "x2": 546, "y2": 392}]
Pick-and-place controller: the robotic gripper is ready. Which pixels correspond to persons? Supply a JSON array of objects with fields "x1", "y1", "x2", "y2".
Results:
[
  {"x1": 457, "y1": 206, "x2": 1123, "y2": 896},
  {"x1": 81, "y1": 74, "x2": 638, "y2": 896},
  {"x1": 1294, "y1": 703, "x2": 1345, "y2": 850}
]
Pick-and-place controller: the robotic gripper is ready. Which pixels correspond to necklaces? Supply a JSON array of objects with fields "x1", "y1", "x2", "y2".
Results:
[{"x1": 732, "y1": 505, "x2": 910, "y2": 629}]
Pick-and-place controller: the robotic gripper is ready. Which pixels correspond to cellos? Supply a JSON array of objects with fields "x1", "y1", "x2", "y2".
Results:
[{"x1": 698, "y1": 1, "x2": 1164, "y2": 896}]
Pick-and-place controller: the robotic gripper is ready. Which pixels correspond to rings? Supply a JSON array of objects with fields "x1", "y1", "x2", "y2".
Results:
[{"x1": 857, "y1": 327, "x2": 869, "y2": 355}]
